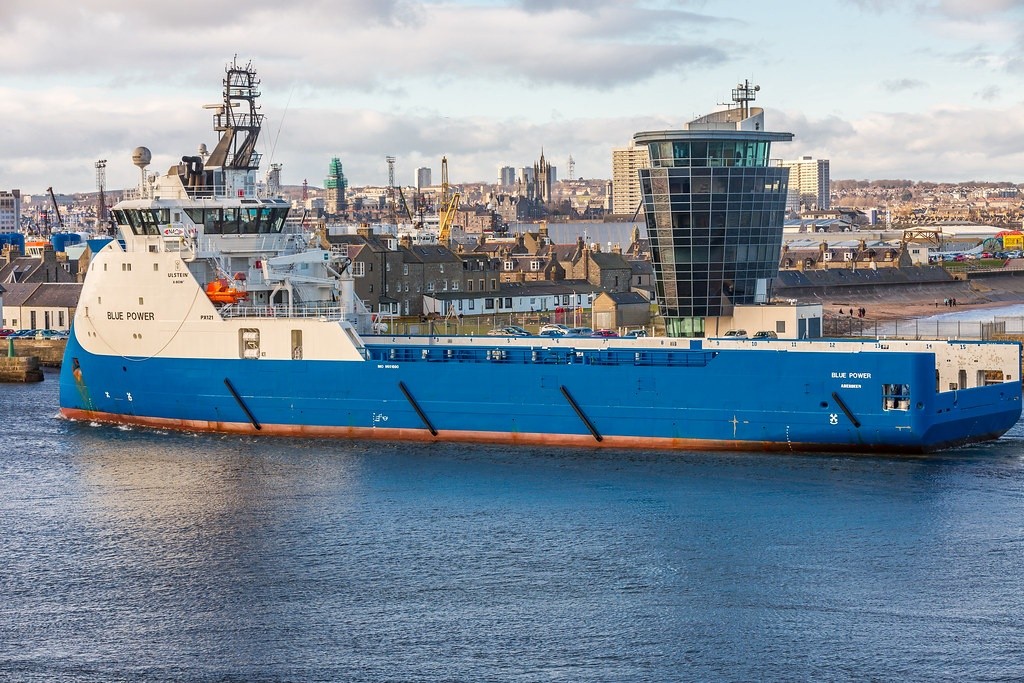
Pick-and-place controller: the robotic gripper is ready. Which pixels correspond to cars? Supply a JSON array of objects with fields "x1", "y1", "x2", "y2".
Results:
[
  {"x1": 724, "y1": 328, "x2": 747, "y2": 340},
  {"x1": 562, "y1": 327, "x2": 593, "y2": 337},
  {"x1": 623, "y1": 330, "x2": 649, "y2": 339},
  {"x1": 922, "y1": 240, "x2": 1024, "y2": 265},
  {"x1": 592, "y1": 330, "x2": 620, "y2": 339},
  {"x1": 0, "y1": 324, "x2": 69, "y2": 341},
  {"x1": 487, "y1": 323, "x2": 568, "y2": 338},
  {"x1": 753, "y1": 329, "x2": 778, "y2": 341}
]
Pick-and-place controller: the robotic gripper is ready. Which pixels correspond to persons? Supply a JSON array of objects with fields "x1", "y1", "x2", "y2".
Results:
[
  {"x1": 953, "y1": 297, "x2": 958, "y2": 307},
  {"x1": 418, "y1": 311, "x2": 429, "y2": 323},
  {"x1": 950, "y1": 297, "x2": 953, "y2": 308},
  {"x1": 267, "y1": 211, "x2": 273, "y2": 222},
  {"x1": 944, "y1": 296, "x2": 949, "y2": 307},
  {"x1": 225, "y1": 215, "x2": 231, "y2": 223},
  {"x1": 839, "y1": 307, "x2": 844, "y2": 315},
  {"x1": 936, "y1": 298, "x2": 940, "y2": 309},
  {"x1": 857, "y1": 306, "x2": 868, "y2": 319},
  {"x1": 459, "y1": 312, "x2": 465, "y2": 324},
  {"x1": 850, "y1": 308, "x2": 854, "y2": 317}
]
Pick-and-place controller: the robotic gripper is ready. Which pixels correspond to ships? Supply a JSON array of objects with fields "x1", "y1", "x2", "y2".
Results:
[{"x1": 57, "y1": 51, "x2": 1024, "y2": 453}]
